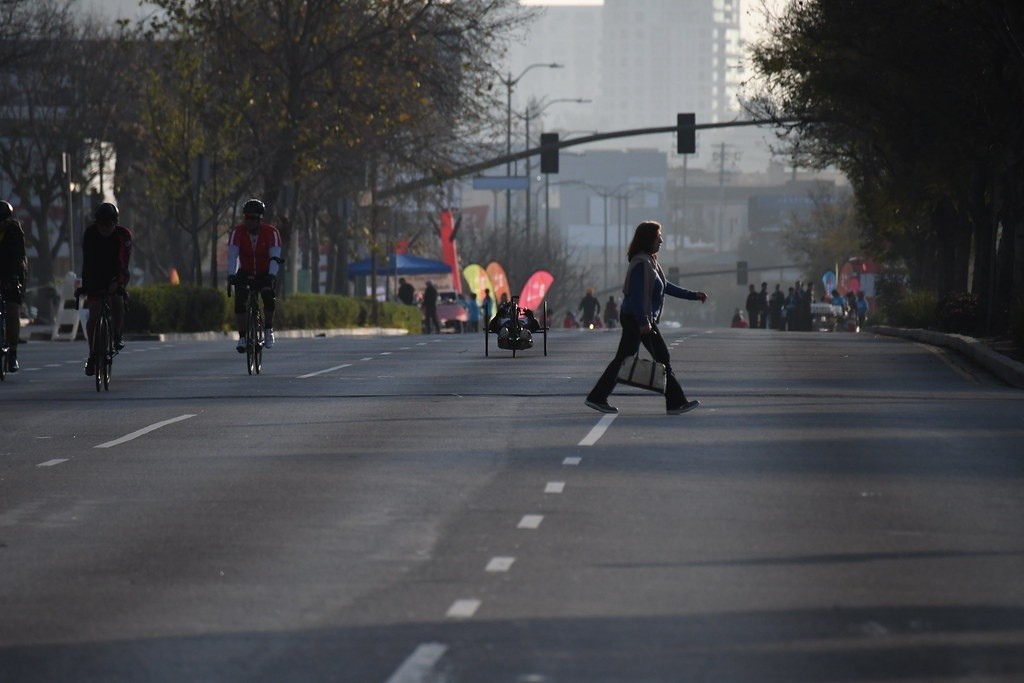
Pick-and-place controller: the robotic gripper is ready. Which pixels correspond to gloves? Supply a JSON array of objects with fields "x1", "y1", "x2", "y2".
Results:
[
  {"x1": 227, "y1": 274, "x2": 237, "y2": 285},
  {"x1": 497, "y1": 306, "x2": 506, "y2": 316},
  {"x1": 525, "y1": 309, "x2": 534, "y2": 319}
]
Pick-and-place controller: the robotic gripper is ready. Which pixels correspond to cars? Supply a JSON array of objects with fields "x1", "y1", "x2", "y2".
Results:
[{"x1": 415, "y1": 293, "x2": 468, "y2": 334}]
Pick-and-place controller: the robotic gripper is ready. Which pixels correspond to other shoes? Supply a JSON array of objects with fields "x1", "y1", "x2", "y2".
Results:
[
  {"x1": 584, "y1": 397, "x2": 618, "y2": 413},
  {"x1": 520, "y1": 329, "x2": 532, "y2": 341},
  {"x1": 236, "y1": 336, "x2": 246, "y2": 354},
  {"x1": 84, "y1": 358, "x2": 96, "y2": 376},
  {"x1": 113, "y1": 336, "x2": 125, "y2": 348},
  {"x1": 8, "y1": 352, "x2": 20, "y2": 373},
  {"x1": 500, "y1": 327, "x2": 509, "y2": 337},
  {"x1": 666, "y1": 399, "x2": 700, "y2": 415},
  {"x1": 264, "y1": 327, "x2": 275, "y2": 348}
]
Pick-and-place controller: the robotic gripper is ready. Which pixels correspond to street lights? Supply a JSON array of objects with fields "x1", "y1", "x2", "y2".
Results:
[
  {"x1": 488, "y1": 97, "x2": 592, "y2": 281},
  {"x1": 491, "y1": 130, "x2": 600, "y2": 275},
  {"x1": 461, "y1": 59, "x2": 565, "y2": 287},
  {"x1": 571, "y1": 179, "x2": 658, "y2": 298}
]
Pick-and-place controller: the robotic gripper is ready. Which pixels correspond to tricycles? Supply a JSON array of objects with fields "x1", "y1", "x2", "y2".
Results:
[{"x1": 482, "y1": 297, "x2": 549, "y2": 357}]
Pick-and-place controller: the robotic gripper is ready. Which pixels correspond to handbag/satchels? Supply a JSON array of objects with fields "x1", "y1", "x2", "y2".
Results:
[{"x1": 614, "y1": 327, "x2": 666, "y2": 393}]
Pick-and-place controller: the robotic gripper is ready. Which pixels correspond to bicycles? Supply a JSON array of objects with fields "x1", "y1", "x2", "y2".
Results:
[
  {"x1": 226, "y1": 276, "x2": 278, "y2": 376},
  {"x1": 0, "y1": 278, "x2": 23, "y2": 381},
  {"x1": 75, "y1": 285, "x2": 122, "y2": 393}
]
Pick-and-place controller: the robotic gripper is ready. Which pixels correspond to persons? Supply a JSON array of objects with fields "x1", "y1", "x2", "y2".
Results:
[
  {"x1": 397, "y1": 277, "x2": 416, "y2": 306},
  {"x1": 478, "y1": 289, "x2": 493, "y2": 332},
  {"x1": 0, "y1": 199, "x2": 26, "y2": 370},
  {"x1": 228, "y1": 199, "x2": 284, "y2": 352},
  {"x1": 732, "y1": 281, "x2": 868, "y2": 336},
  {"x1": 491, "y1": 300, "x2": 542, "y2": 339},
  {"x1": 79, "y1": 204, "x2": 132, "y2": 376},
  {"x1": 422, "y1": 279, "x2": 442, "y2": 334},
  {"x1": 466, "y1": 293, "x2": 480, "y2": 334},
  {"x1": 538, "y1": 287, "x2": 621, "y2": 331},
  {"x1": 584, "y1": 221, "x2": 708, "y2": 415}
]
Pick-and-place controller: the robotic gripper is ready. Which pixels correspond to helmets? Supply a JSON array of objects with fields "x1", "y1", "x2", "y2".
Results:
[
  {"x1": 95, "y1": 203, "x2": 119, "y2": 220},
  {"x1": 242, "y1": 199, "x2": 265, "y2": 212},
  {"x1": 0, "y1": 200, "x2": 14, "y2": 219}
]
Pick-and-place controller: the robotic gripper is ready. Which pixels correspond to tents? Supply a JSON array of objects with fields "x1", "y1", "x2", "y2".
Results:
[{"x1": 346, "y1": 248, "x2": 452, "y2": 304}]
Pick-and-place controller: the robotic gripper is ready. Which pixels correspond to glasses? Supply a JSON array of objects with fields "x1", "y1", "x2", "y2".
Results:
[
  {"x1": 93, "y1": 221, "x2": 116, "y2": 227},
  {"x1": 244, "y1": 215, "x2": 259, "y2": 220}
]
